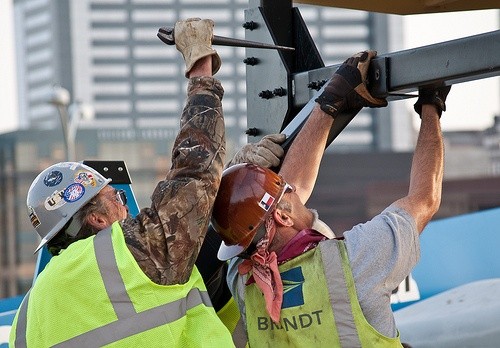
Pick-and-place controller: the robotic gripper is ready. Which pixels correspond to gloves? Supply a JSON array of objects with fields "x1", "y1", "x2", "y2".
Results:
[
  {"x1": 314, "y1": 50, "x2": 388, "y2": 119},
  {"x1": 228, "y1": 133, "x2": 286, "y2": 168},
  {"x1": 413, "y1": 83, "x2": 452, "y2": 119},
  {"x1": 174, "y1": 18, "x2": 222, "y2": 79}
]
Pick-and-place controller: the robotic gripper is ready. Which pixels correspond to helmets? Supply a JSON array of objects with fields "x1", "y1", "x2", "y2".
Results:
[
  {"x1": 208, "y1": 162, "x2": 288, "y2": 261},
  {"x1": 26, "y1": 161, "x2": 112, "y2": 254}
]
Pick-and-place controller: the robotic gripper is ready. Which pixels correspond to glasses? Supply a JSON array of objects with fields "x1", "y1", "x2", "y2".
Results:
[{"x1": 98, "y1": 188, "x2": 127, "y2": 206}]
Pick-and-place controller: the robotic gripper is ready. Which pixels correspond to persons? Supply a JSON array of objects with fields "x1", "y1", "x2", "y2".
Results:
[
  {"x1": 7, "y1": 16, "x2": 236, "y2": 348},
  {"x1": 210, "y1": 49, "x2": 454, "y2": 348}
]
